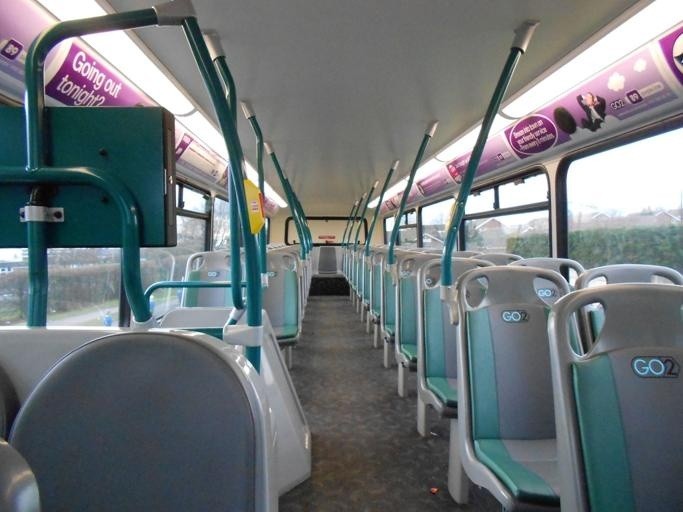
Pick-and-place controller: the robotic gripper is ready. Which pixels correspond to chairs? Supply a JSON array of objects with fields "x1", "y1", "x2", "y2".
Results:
[{"x1": 318, "y1": 246, "x2": 337, "y2": 276}]
